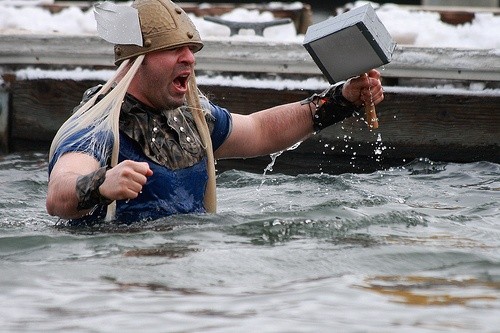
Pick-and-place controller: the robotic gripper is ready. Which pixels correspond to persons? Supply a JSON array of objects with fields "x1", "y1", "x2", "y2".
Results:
[{"x1": 46, "y1": 1, "x2": 384, "y2": 228}]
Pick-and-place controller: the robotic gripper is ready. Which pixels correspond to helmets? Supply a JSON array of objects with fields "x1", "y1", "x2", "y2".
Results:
[{"x1": 94, "y1": 0, "x2": 204, "y2": 65}]
